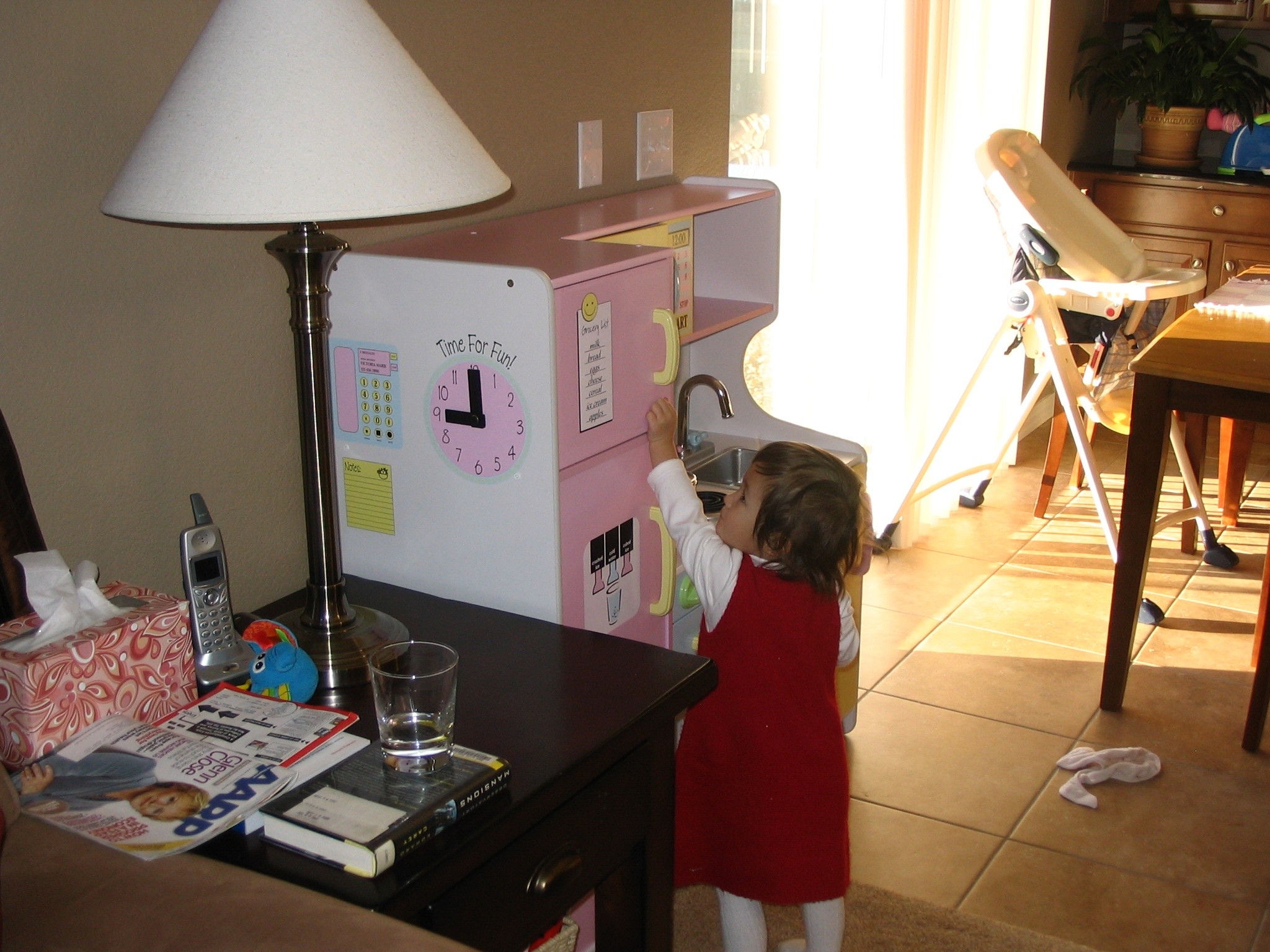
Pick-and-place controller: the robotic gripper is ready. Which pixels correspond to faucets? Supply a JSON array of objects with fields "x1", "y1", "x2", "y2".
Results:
[{"x1": 675, "y1": 371, "x2": 735, "y2": 465}]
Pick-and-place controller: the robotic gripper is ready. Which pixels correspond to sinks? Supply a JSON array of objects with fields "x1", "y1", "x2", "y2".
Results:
[{"x1": 684, "y1": 444, "x2": 762, "y2": 490}]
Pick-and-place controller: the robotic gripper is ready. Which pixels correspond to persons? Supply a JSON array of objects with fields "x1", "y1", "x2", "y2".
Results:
[{"x1": 642, "y1": 396, "x2": 864, "y2": 952}]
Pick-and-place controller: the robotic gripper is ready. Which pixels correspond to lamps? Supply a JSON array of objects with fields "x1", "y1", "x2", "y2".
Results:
[{"x1": 104, "y1": 2, "x2": 521, "y2": 704}]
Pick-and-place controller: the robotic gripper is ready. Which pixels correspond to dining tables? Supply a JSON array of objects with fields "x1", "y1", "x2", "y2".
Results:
[{"x1": 1102, "y1": 251, "x2": 1270, "y2": 750}]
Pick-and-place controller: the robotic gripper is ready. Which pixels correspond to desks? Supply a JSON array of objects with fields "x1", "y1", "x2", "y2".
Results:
[{"x1": 154, "y1": 576, "x2": 717, "y2": 950}]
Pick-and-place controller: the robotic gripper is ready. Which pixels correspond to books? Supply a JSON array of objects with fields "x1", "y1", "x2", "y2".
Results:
[{"x1": 7, "y1": 680, "x2": 512, "y2": 882}]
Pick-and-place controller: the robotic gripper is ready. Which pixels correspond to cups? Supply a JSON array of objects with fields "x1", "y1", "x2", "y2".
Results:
[{"x1": 365, "y1": 641, "x2": 461, "y2": 752}]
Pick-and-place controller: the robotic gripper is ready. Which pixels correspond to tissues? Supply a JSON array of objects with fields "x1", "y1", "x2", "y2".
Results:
[{"x1": 1, "y1": 545, "x2": 200, "y2": 773}]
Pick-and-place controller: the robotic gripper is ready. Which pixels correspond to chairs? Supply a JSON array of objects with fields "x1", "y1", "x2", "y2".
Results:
[{"x1": 875, "y1": 119, "x2": 1239, "y2": 624}]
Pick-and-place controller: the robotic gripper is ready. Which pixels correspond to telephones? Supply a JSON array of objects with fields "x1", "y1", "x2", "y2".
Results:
[{"x1": 182, "y1": 491, "x2": 259, "y2": 689}]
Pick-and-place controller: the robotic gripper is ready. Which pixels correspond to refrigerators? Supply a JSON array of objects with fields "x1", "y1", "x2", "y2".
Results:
[{"x1": 332, "y1": 232, "x2": 679, "y2": 652}]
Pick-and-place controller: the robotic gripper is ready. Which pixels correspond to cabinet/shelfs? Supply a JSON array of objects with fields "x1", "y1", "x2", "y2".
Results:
[{"x1": 1075, "y1": 172, "x2": 1270, "y2": 339}]
